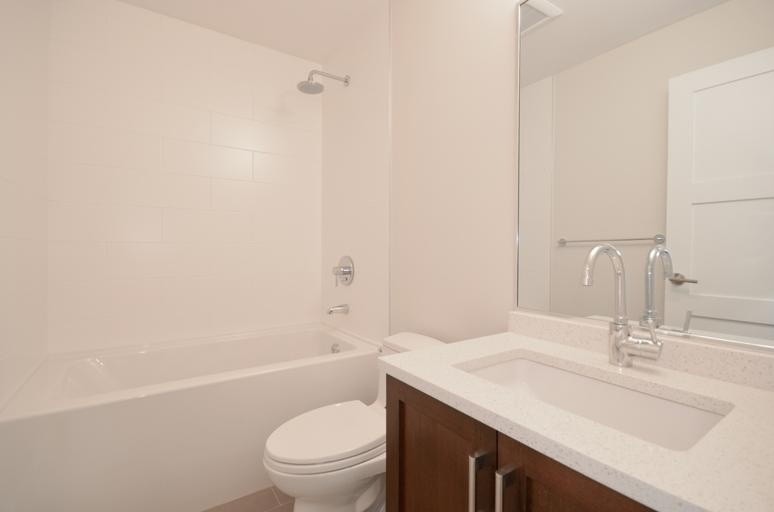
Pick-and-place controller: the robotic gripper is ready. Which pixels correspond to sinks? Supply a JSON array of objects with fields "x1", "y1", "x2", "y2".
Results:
[{"x1": 453, "y1": 346, "x2": 736, "y2": 455}]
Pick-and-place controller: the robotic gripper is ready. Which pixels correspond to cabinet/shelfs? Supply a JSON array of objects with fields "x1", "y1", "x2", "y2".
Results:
[{"x1": 385, "y1": 373, "x2": 660, "y2": 511}]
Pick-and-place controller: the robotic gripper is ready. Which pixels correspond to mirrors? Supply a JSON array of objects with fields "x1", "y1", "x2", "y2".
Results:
[{"x1": 516, "y1": 1, "x2": 774, "y2": 354}]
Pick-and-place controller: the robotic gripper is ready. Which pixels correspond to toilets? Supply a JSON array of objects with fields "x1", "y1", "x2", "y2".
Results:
[{"x1": 264, "y1": 334, "x2": 445, "y2": 511}]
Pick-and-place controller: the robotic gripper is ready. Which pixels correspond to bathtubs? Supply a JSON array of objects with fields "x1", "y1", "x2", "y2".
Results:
[{"x1": 1, "y1": 324, "x2": 381, "y2": 512}]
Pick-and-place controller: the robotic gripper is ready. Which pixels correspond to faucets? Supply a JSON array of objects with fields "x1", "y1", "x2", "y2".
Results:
[
  {"x1": 583, "y1": 244, "x2": 664, "y2": 367},
  {"x1": 639, "y1": 245, "x2": 673, "y2": 331},
  {"x1": 325, "y1": 303, "x2": 349, "y2": 317}
]
